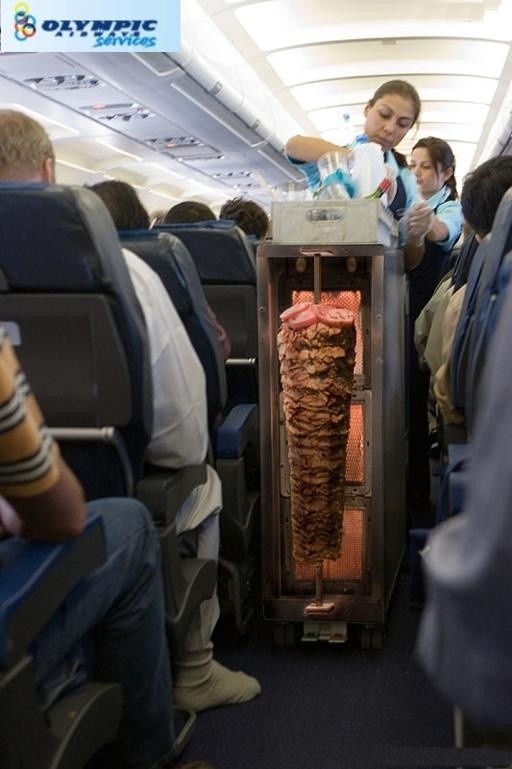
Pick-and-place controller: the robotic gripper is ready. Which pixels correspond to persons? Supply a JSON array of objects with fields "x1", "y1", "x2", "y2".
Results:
[
  {"x1": 1, "y1": 107, "x2": 510, "y2": 768},
  {"x1": 285, "y1": 80, "x2": 430, "y2": 276}
]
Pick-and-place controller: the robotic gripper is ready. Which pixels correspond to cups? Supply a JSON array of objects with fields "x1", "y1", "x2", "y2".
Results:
[
  {"x1": 317, "y1": 150, "x2": 353, "y2": 199},
  {"x1": 355, "y1": 142, "x2": 388, "y2": 207}
]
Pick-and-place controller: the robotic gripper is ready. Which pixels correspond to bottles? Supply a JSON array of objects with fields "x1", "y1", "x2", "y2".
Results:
[{"x1": 363, "y1": 178, "x2": 391, "y2": 200}]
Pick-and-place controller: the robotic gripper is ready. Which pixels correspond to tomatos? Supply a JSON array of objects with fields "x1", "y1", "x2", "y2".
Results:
[{"x1": 279, "y1": 302, "x2": 355, "y2": 329}]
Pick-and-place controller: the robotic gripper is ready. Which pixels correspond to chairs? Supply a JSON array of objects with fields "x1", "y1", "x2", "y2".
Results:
[
  {"x1": 0, "y1": 180, "x2": 270, "y2": 769},
  {"x1": 407, "y1": 184, "x2": 512, "y2": 766}
]
